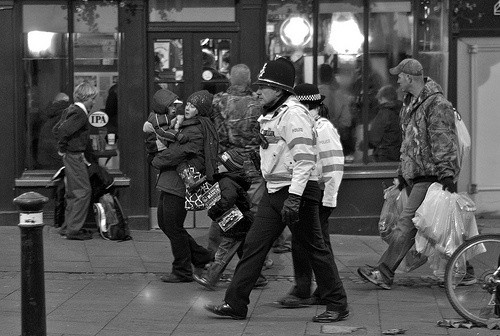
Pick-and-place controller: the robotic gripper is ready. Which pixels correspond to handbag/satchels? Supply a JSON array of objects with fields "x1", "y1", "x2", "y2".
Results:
[{"x1": 177, "y1": 163, "x2": 213, "y2": 211}]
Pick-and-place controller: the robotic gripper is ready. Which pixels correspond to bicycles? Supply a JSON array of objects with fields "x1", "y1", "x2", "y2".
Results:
[{"x1": 443, "y1": 233, "x2": 500, "y2": 328}]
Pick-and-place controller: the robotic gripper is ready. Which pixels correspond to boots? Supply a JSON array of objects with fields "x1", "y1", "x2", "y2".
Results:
[{"x1": 179, "y1": 168, "x2": 207, "y2": 193}]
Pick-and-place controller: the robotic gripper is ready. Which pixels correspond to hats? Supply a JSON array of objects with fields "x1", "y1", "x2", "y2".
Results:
[
  {"x1": 173, "y1": 99, "x2": 183, "y2": 108},
  {"x1": 219, "y1": 150, "x2": 244, "y2": 172},
  {"x1": 294, "y1": 84, "x2": 325, "y2": 104},
  {"x1": 252, "y1": 54, "x2": 296, "y2": 97},
  {"x1": 389, "y1": 59, "x2": 423, "y2": 75},
  {"x1": 186, "y1": 90, "x2": 213, "y2": 116}
]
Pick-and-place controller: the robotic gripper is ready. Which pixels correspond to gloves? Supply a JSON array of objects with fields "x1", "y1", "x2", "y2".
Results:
[
  {"x1": 398, "y1": 175, "x2": 408, "y2": 191},
  {"x1": 175, "y1": 132, "x2": 189, "y2": 144},
  {"x1": 281, "y1": 195, "x2": 301, "y2": 225},
  {"x1": 442, "y1": 177, "x2": 456, "y2": 193}
]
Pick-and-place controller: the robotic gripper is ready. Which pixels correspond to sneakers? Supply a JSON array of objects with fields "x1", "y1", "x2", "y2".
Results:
[
  {"x1": 439, "y1": 267, "x2": 476, "y2": 287},
  {"x1": 357, "y1": 266, "x2": 392, "y2": 290}
]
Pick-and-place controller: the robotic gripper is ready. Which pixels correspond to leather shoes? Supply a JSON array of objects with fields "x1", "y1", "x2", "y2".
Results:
[
  {"x1": 205, "y1": 301, "x2": 245, "y2": 319},
  {"x1": 313, "y1": 306, "x2": 349, "y2": 322}
]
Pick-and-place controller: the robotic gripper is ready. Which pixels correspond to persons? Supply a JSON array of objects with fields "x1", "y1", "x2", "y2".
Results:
[
  {"x1": 192, "y1": 149, "x2": 268, "y2": 290},
  {"x1": 357, "y1": 58, "x2": 477, "y2": 290},
  {"x1": 52, "y1": 82, "x2": 98, "y2": 240},
  {"x1": 203, "y1": 57, "x2": 350, "y2": 322},
  {"x1": 360, "y1": 85, "x2": 401, "y2": 162},
  {"x1": 318, "y1": 63, "x2": 352, "y2": 142},
  {"x1": 144, "y1": 89, "x2": 206, "y2": 193},
  {"x1": 275, "y1": 83, "x2": 344, "y2": 307},
  {"x1": 209, "y1": 63, "x2": 273, "y2": 270},
  {"x1": 38, "y1": 92, "x2": 70, "y2": 229},
  {"x1": 142, "y1": 89, "x2": 214, "y2": 283},
  {"x1": 104, "y1": 84, "x2": 118, "y2": 142}
]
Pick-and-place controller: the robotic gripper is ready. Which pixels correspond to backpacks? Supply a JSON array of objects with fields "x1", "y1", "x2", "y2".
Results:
[
  {"x1": 453, "y1": 107, "x2": 471, "y2": 156},
  {"x1": 93, "y1": 193, "x2": 132, "y2": 242}
]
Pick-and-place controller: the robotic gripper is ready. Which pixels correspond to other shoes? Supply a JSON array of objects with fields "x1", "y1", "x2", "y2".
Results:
[
  {"x1": 281, "y1": 293, "x2": 315, "y2": 306},
  {"x1": 66, "y1": 231, "x2": 92, "y2": 240},
  {"x1": 192, "y1": 273, "x2": 213, "y2": 290},
  {"x1": 160, "y1": 273, "x2": 181, "y2": 283},
  {"x1": 255, "y1": 279, "x2": 269, "y2": 288},
  {"x1": 262, "y1": 258, "x2": 274, "y2": 271}
]
